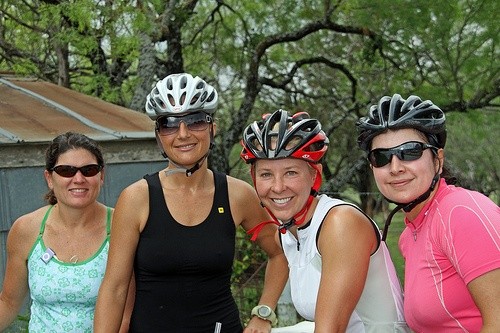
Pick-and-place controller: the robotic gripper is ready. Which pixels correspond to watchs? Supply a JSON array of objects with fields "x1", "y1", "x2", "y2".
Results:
[{"x1": 250, "y1": 305, "x2": 278, "y2": 328}]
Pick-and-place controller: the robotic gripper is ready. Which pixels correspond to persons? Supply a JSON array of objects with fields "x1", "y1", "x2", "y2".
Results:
[
  {"x1": 357, "y1": 94, "x2": 500, "y2": 333},
  {"x1": 0, "y1": 132, "x2": 136, "y2": 333},
  {"x1": 94, "y1": 74, "x2": 286, "y2": 333},
  {"x1": 241, "y1": 108, "x2": 410, "y2": 333}
]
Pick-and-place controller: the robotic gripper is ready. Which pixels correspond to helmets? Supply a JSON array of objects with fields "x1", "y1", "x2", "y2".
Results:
[
  {"x1": 355, "y1": 94, "x2": 447, "y2": 151},
  {"x1": 240, "y1": 109, "x2": 329, "y2": 164},
  {"x1": 145, "y1": 73, "x2": 218, "y2": 121}
]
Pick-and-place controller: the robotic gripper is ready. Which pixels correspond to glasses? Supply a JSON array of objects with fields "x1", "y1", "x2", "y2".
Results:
[
  {"x1": 50, "y1": 164, "x2": 104, "y2": 178},
  {"x1": 154, "y1": 112, "x2": 214, "y2": 136},
  {"x1": 367, "y1": 141, "x2": 439, "y2": 168}
]
